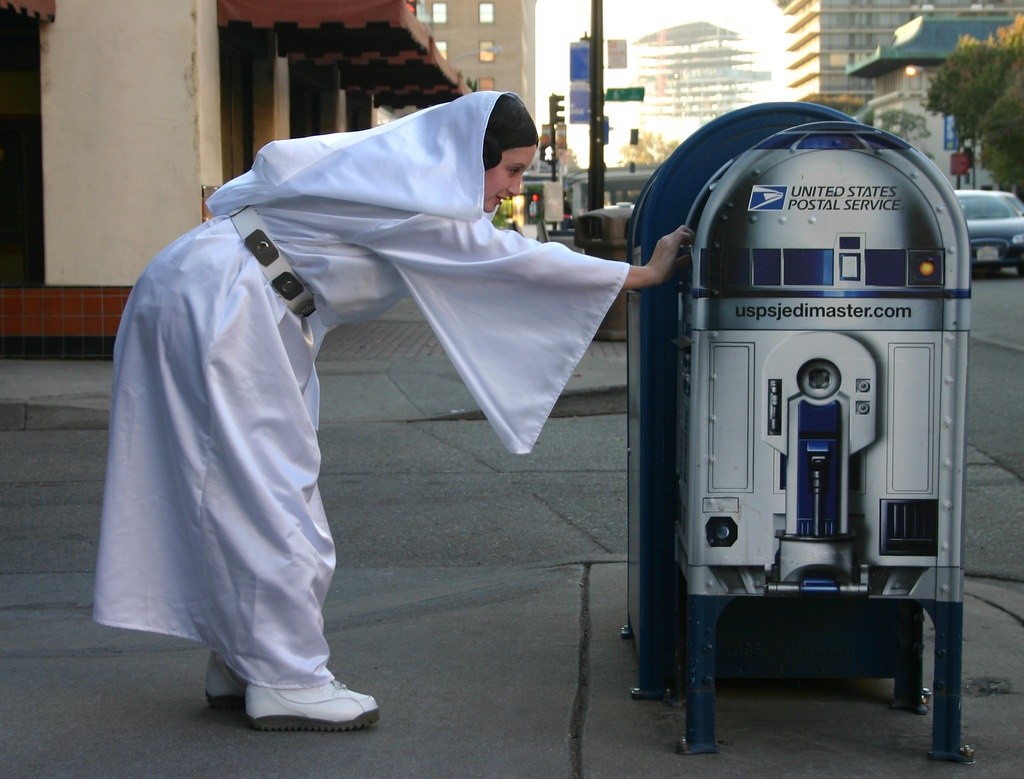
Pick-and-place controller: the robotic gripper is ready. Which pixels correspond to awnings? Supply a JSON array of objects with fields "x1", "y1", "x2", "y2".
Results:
[{"x1": 217, "y1": 0, "x2": 475, "y2": 108}]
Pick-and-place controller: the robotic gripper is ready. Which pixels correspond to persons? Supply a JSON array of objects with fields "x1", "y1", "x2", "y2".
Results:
[{"x1": 91, "y1": 91, "x2": 696, "y2": 731}]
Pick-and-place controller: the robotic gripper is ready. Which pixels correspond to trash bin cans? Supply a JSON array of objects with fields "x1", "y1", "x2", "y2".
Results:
[
  {"x1": 622, "y1": 100, "x2": 939, "y2": 715},
  {"x1": 674, "y1": 122, "x2": 973, "y2": 598},
  {"x1": 575, "y1": 208, "x2": 635, "y2": 341}
]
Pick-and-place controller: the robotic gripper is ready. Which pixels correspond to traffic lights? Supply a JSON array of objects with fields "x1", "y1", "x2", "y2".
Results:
[
  {"x1": 552, "y1": 94, "x2": 566, "y2": 124},
  {"x1": 527, "y1": 190, "x2": 539, "y2": 202},
  {"x1": 540, "y1": 144, "x2": 554, "y2": 162}
]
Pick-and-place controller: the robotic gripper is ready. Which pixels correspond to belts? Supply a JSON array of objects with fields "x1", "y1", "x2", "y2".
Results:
[{"x1": 228, "y1": 204, "x2": 316, "y2": 316}]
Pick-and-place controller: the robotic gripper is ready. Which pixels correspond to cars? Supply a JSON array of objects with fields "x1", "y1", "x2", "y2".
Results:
[
  {"x1": 560, "y1": 210, "x2": 575, "y2": 229},
  {"x1": 954, "y1": 188, "x2": 1024, "y2": 279}
]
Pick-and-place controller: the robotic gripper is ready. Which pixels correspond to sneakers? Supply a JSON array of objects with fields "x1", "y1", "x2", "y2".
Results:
[
  {"x1": 205, "y1": 661, "x2": 248, "y2": 710},
  {"x1": 245, "y1": 681, "x2": 380, "y2": 732}
]
